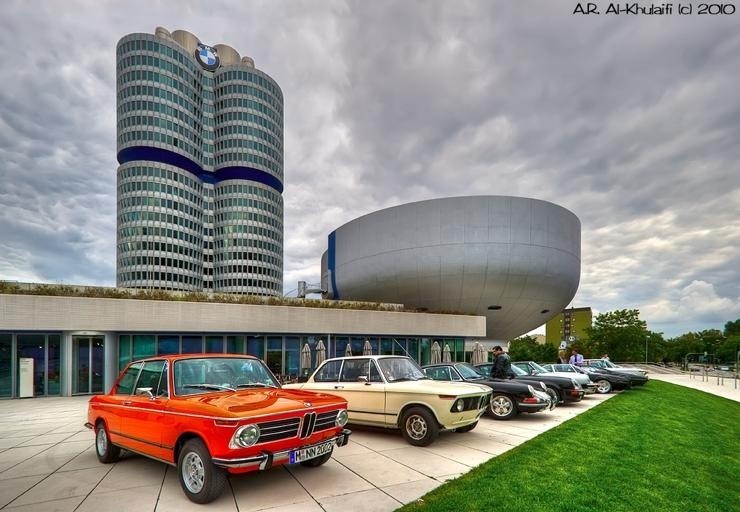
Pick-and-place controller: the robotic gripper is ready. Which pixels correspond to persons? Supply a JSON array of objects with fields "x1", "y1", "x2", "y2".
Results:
[
  {"x1": 601, "y1": 353, "x2": 610, "y2": 361},
  {"x1": 556, "y1": 349, "x2": 567, "y2": 365},
  {"x1": 489, "y1": 346, "x2": 514, "y2": 379},
  {"x1": 569, "y1": 349, "x2": 584, "y2": 367}
]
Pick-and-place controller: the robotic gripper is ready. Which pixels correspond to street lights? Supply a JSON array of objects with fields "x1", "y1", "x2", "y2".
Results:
[{"x1": 645, "y1": 334, "x2": 650, "y2": 364}]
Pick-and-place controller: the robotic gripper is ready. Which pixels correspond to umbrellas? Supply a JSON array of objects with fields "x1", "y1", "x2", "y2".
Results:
[
  {"x1": 479, "y1": 344, "x2": 488, "y2": 363},
  {"x1": 345, "y1": 344, "x2": 354, "y2": 369},
  {"x1": 472, "y1": 341, "x2": 482, "y2": 366},
  {"x1": 363, "y1": 340, "x2": 373, "y2": 375},
  {"x1": 314, "y1": 340, "x2": 328, "y2": 380},
  {"x1": 430, "y1": 342, "x2": 442, "y2": 378},
  {"x1": 300, "y1": 343, "x2": 312, "y2": 380},
  {"x1": 443, "y1": 344, "x2": 451, "y2": 364}
]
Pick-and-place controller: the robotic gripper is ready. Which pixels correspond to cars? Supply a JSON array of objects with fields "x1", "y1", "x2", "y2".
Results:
[
  {"x1": 418, "y1": 361, "x2": 556, "y2": 422},
  {"x1": 509, "y1": 357, "x2": 654, "y2": 405},
  {"x1": 280, "y1": 354, "x2": 495, "y2": 448},
  {"x1": 82, "y1": 352, "x2": 352, "y2": 507}
]
